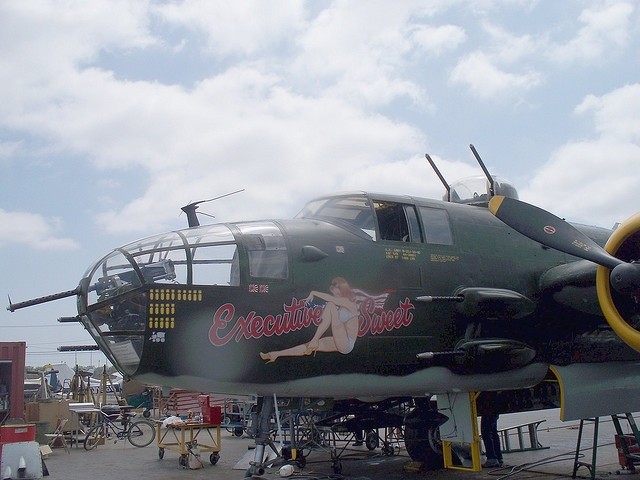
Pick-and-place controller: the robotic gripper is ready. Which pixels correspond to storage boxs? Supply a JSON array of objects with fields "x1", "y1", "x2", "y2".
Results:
[{"x1": 39, "y1": 445, "x2": 52, "y2": 459}]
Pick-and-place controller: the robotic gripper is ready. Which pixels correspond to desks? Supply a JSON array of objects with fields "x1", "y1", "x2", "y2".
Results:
[
  {"x1": 152, "y1": 420, "x2": 221, "y2": 469},
  {"x1": 615, "y1": 433, "x2": 640, "y2": 471}
]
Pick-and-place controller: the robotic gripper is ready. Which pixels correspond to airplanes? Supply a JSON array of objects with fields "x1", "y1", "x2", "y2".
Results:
[{"x1": 5, "y1": 147, "x2": 640, "y2": 474}]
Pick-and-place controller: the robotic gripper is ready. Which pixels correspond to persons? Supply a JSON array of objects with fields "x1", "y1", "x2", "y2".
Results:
[
  {"x1": 260, "y1": 277, "x2": 359, "y2": 364},
  {"x1": 480, "y1": 389, "x2": 504, "y2": 468}
]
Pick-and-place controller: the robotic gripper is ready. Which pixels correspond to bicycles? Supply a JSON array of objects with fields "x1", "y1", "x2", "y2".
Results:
[{"x1": 84, "y1": 402, "x2": 156, "y2": 451}]
